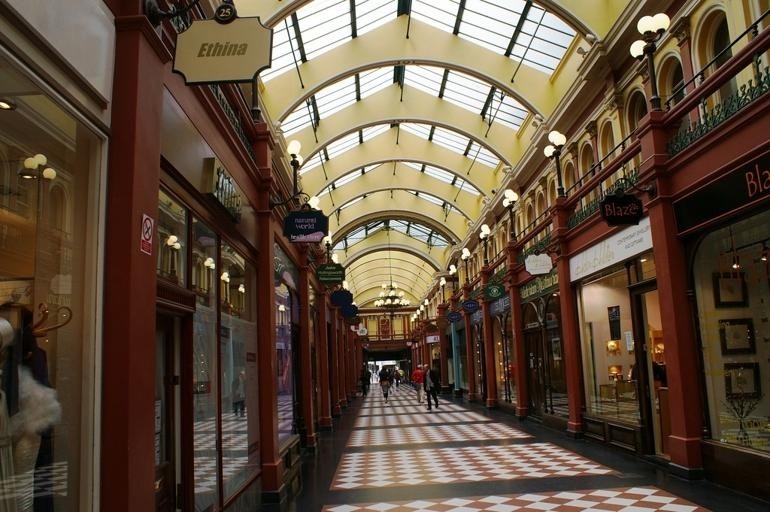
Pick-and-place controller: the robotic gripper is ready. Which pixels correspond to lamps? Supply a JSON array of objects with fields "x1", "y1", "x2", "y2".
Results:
[{"x1": 725, "y1": 240, "x2": 769, "y2": 269}]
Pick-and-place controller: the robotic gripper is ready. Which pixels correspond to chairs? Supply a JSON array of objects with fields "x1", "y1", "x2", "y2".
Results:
[{"x1": 599, "y1": 378, "x2": 638, "y2": 420}]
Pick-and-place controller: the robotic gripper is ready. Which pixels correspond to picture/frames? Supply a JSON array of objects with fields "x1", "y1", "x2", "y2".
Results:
[{"x1": 712, "y1": 270, "x2": 762, "y2": 402}]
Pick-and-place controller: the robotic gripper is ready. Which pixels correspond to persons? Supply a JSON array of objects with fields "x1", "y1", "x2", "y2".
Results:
[
  {"x1": 411, "y1": 363, "x2": 442, "y2": 410},
  {"x1": 359, "y1": 369, "x2": 371, "y2": 398},
  {"x1": 231, "y1": 370, "x2": 247, "y2": 416},
  {"x1": 379, "y1": 365, "x2": 401, "y2": 403}
]
